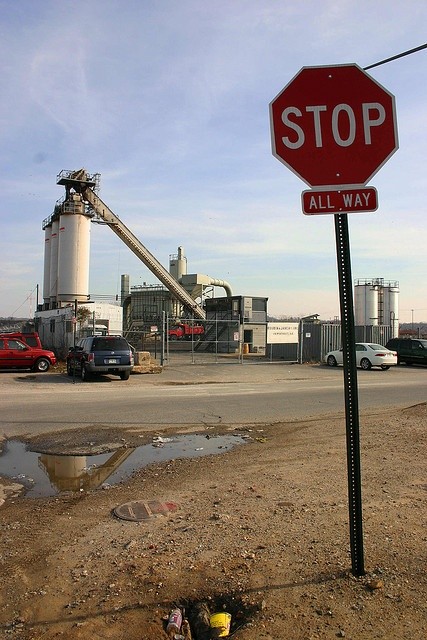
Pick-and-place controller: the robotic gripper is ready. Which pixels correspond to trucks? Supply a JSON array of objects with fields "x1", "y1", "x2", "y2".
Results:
[
  {"x1": 384, "y1": 337, "x2": 427, "y2": 366},
  {"x1": 163, "y1": 323, "x2": 205, "y2": 341}
]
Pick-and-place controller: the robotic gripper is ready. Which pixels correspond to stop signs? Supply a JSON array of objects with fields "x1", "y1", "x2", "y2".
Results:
[{"x1": 268, "y1": 62, "x2": 399, "y2": 190}]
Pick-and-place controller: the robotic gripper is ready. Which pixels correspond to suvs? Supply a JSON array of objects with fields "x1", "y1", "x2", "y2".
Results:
[
  {"x1": 0, "y1": 337, "x2": 56, "y2": 372},
  {"x1": 66, "y1": 335, "x2": 134, "y2": 381}
]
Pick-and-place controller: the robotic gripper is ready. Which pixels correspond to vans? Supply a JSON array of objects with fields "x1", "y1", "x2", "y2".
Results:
[{"x1": 0, "y1": 332, "x2": 43, "y2": 350}]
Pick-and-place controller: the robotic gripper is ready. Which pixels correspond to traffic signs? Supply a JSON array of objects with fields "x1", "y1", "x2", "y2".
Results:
[{"x1": 300, "y1": 185, "x2": 379, "y2": 215}]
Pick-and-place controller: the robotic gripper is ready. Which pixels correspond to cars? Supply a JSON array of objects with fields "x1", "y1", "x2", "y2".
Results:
[{"x1": 322, "y1": 342, "x2": 398, "y2": 371}]
